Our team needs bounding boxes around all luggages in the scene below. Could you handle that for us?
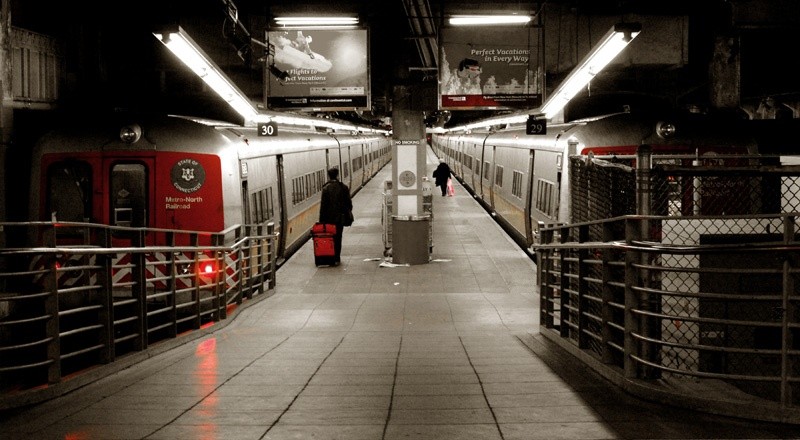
[312,222,337,267]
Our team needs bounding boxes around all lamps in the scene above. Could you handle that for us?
[151,16,394,131]
[423,11,642,134]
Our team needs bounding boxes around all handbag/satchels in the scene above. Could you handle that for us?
[343,210,354,227]
[310,223,336,237]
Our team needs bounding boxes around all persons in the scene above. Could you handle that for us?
[436,159,451,196]
[319,168,353,266]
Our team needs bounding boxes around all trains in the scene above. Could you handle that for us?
[428,115,776,258]
[26,109,388,310]
